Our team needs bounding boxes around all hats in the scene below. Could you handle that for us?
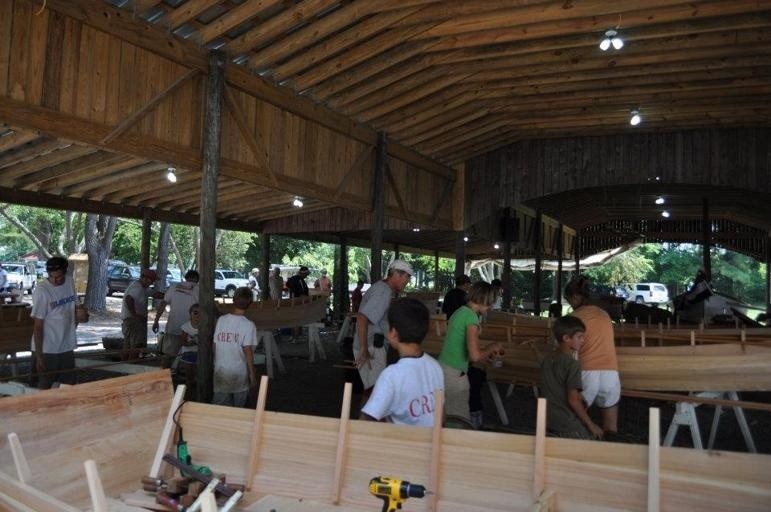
[300,267,310,273]
[251,268,258,273]
[389,260,417,276]
[142,267,156,282]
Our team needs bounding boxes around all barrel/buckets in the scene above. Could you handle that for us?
[177,355,196,399]
[76,309,89,323]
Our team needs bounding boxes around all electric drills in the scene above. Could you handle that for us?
[178,441,212,480]
[368,476,434,512]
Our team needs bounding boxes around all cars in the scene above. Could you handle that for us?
[165,266,190,289]
[613,286,629,299]
[105,263,170,296]
[0,262,38,294]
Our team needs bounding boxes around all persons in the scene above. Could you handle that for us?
[269,266,332,299]
[351,259,507,435]
[152,270,258,408]
[121,269,165,359]
[30,256,89,390]
[537,276,621,441]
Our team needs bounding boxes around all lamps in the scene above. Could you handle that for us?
[596,26,626,54]
[629,104,642,126]
[290,194,307,211]
[167,163,178,187]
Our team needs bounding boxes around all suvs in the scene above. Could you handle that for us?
[214,268,250,299]
[626,281,669,307]
